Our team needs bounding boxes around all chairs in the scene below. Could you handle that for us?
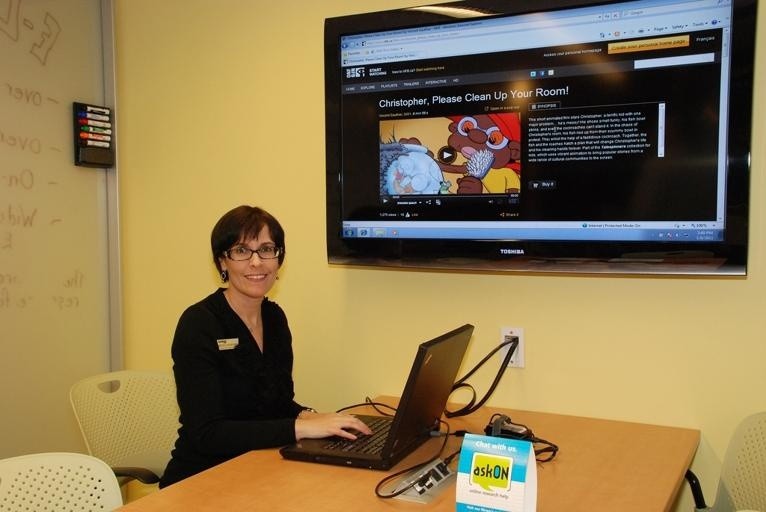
[68,366,183,501]
[686,409,766,510]
[0,452,122,511]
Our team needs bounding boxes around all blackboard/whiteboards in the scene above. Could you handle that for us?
[0,0,137,488]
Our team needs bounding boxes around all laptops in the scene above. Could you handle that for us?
[280,322,476,469]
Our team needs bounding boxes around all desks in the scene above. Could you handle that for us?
[113,392,701,511]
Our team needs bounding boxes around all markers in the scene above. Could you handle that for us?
[77,104,112,148]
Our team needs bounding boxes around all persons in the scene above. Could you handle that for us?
[154,204,372,490]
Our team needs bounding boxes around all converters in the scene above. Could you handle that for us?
[484,419,531,442]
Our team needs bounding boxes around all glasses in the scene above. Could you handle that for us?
[224,245,283,261]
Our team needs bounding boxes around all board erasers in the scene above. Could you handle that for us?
[78,148,114,166]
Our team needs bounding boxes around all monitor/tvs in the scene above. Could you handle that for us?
[323,0,757,278]
